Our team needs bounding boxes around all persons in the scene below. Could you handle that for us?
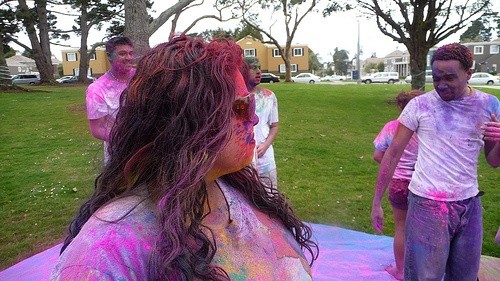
[373,89,426,281]
[49,36,314,281]
[371,43,500,281]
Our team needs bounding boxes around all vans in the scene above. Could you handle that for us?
[361,71,399,84]
[405,69,433,82]
[12,73,41,85]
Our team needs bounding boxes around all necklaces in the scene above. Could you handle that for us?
[108,68,131,83]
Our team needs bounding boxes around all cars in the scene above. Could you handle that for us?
[290,72,321,84]
[56,76,74,83]
[467,71,500,85]
[260,73,281,84]
[61,75,95,83]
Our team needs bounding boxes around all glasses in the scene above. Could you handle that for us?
[232,92,256,123]
